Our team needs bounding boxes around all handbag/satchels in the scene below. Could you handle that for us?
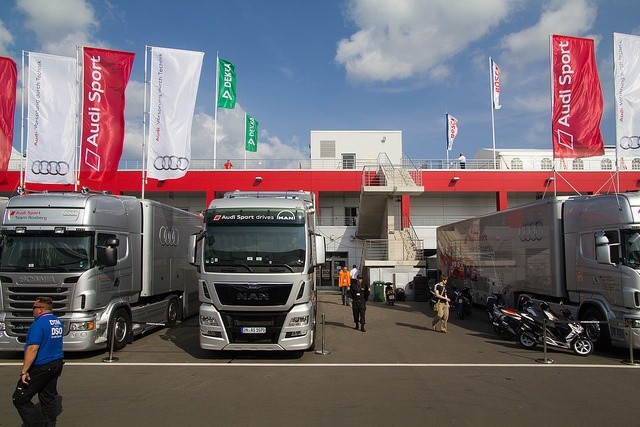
[433,303,439,311]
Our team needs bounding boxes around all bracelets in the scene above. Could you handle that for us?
[19,372,28,375]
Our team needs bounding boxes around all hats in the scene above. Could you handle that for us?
[357,275,363,278]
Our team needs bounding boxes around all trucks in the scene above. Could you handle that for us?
[187,189,326,358]
[436,191,640,349]
[0,185,204,351]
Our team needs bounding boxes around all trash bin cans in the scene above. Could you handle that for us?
[373,281,385,302]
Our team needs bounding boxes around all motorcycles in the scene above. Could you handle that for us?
[487,291,506,318]
[454,285,472,319]
[384,281,397,305]
[515,300,594,356]
[492,297,560,337]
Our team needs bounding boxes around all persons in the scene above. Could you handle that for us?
[224,159,233,168]
[350,264,359,284]
[338,266,351,306]
[458,153,467,168]
[345,274,370,331]
[432,273,451,332]
[12,296,64,426]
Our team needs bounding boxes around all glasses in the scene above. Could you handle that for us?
[33,306,42,309]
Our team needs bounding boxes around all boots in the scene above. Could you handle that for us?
[355,322,359,329]
[361,324,366,332]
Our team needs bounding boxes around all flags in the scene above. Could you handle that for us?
[551,34,605,158]
[0,56,18,183]
[447,114,459,149]
[78,47,136,191]
[492,60,502,109]
[146,46,205,180]
[613,32,640,158]
[27,51,78,191]
[245,112,259,151]
[217,57,236,109]
